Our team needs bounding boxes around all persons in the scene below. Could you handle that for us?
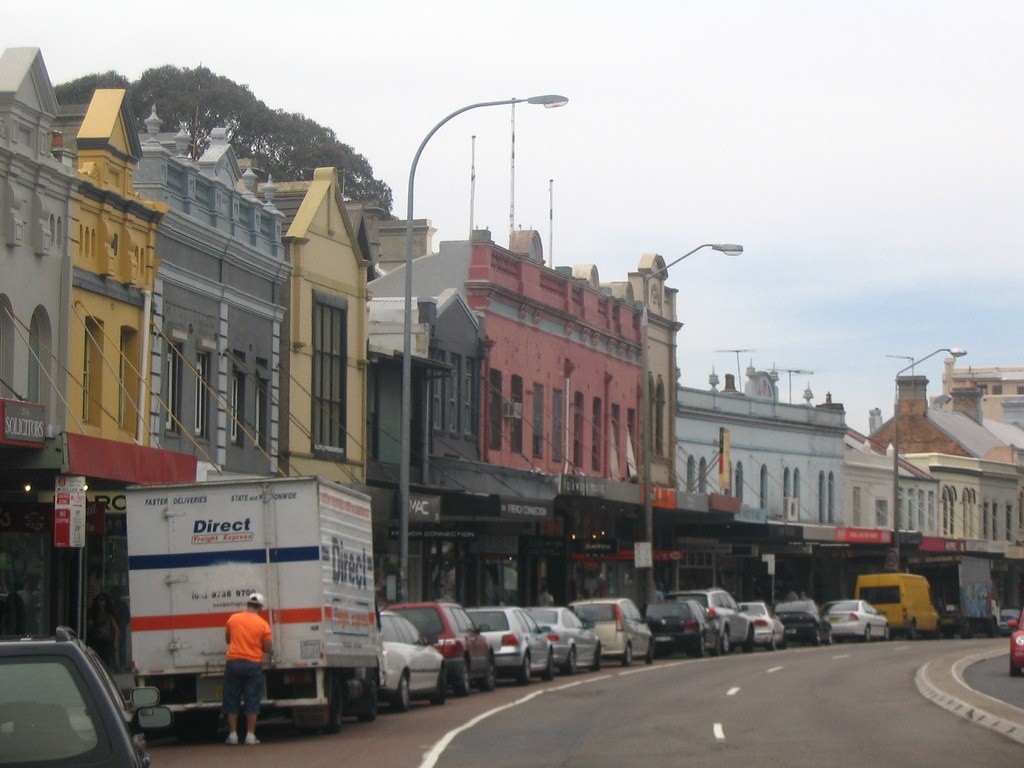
[86,592,120,670]
[535,587,554,607]
[221,592,272,745]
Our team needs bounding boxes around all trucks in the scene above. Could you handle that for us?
[123,476,387,744]
[927,556,999,639]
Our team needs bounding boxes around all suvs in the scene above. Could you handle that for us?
[387,603,498,698]
[0,626,151,768]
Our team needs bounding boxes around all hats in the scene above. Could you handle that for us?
[247,593,263,606]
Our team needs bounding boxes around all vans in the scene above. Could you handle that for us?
[856,573,941,640]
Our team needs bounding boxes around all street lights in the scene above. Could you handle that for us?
[893,348,968,550]
[644,244,743,607]
[885,355,914,375]
[400,95,569,606]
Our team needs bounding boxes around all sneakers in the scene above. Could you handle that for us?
[245,736,260,744]
[226,736,238,744]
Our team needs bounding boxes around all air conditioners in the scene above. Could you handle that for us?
[504,402,522,419]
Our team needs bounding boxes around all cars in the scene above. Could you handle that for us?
[1009,605,1024,678]
[377,610,447,713]
[1000,608,1020,637]
[738,602,788,651]
[642,601,724,658]
[774,599,836,647]
[526,607,602,677]
[467,607,556,686]
[821,600,890,641]
[662,586,754,654]
[568,597,657,668]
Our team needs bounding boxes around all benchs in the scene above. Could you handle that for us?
[0,702,95,764]
